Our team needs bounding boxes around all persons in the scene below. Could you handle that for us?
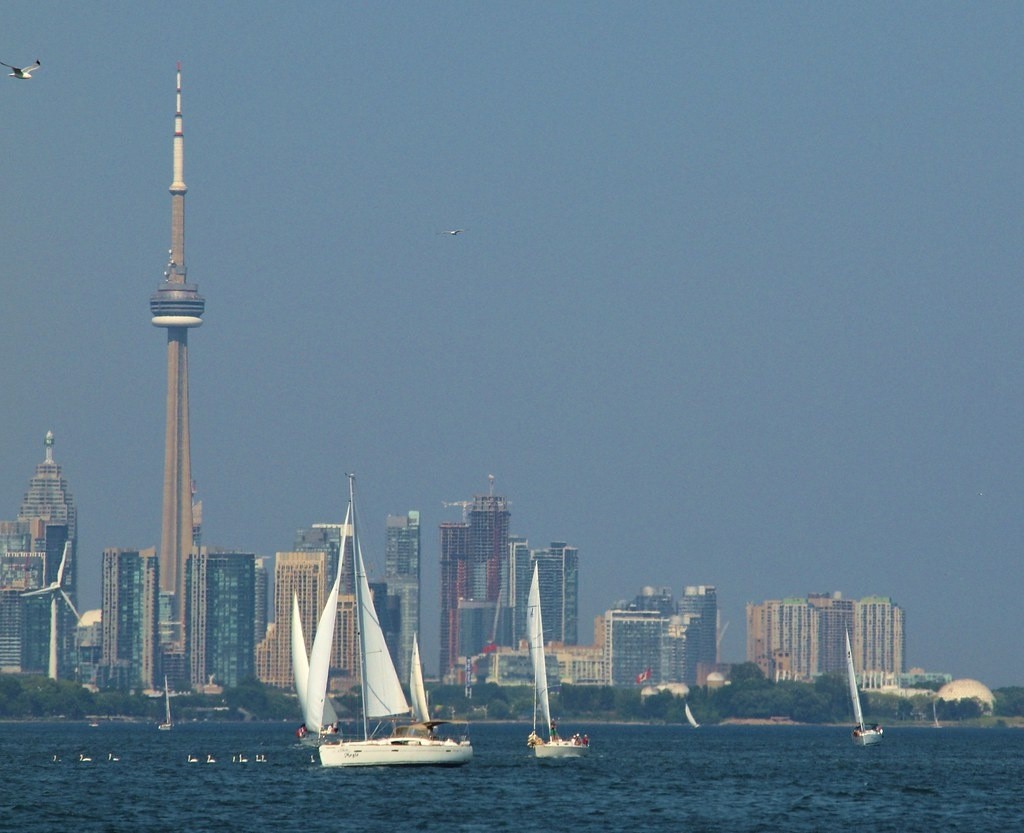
[573,733,589,747]
[550,722,557,741]
[853,724,883,738]
[297,717,338,739]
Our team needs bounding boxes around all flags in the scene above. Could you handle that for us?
[637,668,651,684]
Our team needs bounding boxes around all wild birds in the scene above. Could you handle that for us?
[0,59,40,79]
[436,229,463,236]
[50,753,316,767]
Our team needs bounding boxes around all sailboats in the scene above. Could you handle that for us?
[845,627,883,746]
[290,475,592,768]
[158,675,175,730]
[685,703,700,727]
